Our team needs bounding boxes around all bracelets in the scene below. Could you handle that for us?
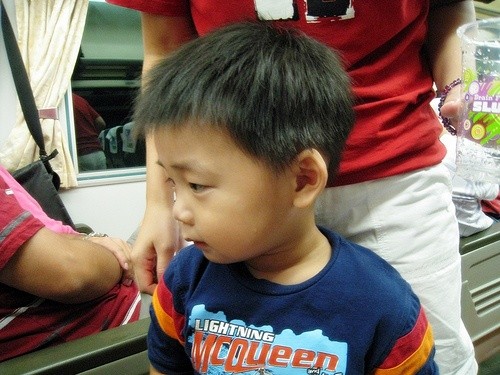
[438,76,468,137]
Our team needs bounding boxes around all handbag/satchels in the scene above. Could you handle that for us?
[15,155,77,231]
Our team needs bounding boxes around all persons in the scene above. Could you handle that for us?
[71,90,108,172]
[1,175,135,301]
[122,17,444,375]
[99,0,500,375]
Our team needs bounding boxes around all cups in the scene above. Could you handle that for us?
[455,17,500,182]
